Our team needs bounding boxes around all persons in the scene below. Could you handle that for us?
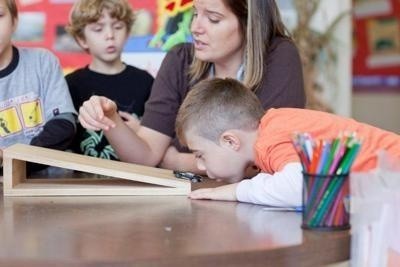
[0,0,79,185]
[64,0,154,174]
[175,77,400,208]
[78,0,306,180]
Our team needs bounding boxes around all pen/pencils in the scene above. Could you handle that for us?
[291,129,366,230]
